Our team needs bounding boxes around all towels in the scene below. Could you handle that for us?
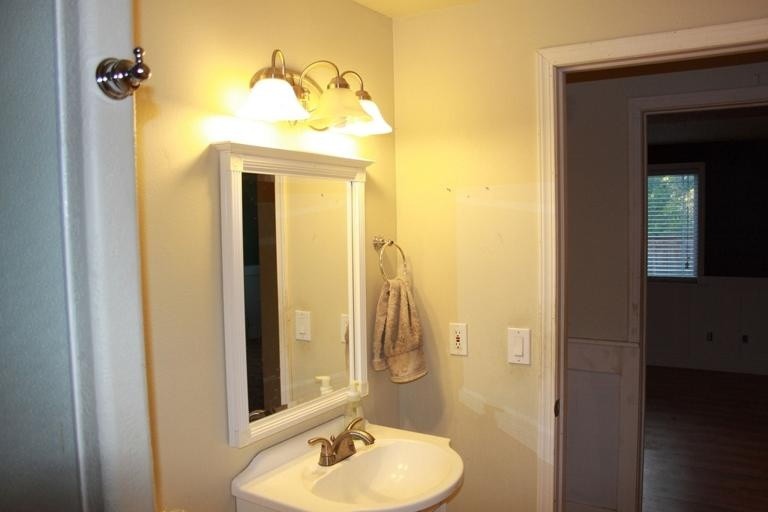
[372,274,431,382]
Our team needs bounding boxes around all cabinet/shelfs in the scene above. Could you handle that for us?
[692,276,741,376]
[646,276,692,370]
[740,276,766,378]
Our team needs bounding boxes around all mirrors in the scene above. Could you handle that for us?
[211,141,377,450]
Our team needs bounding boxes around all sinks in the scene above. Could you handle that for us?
[309,438,453,508]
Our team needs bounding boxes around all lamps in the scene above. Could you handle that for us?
[240,48,393,138]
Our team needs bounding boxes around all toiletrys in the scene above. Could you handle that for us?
[343,381,366,432]
[314,375,334,396]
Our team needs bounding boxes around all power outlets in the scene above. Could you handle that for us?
[448,324,468,357]
[339,313,347,342]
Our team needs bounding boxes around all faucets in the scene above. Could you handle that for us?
[308,417,375,465]
[249,409,273,423]
